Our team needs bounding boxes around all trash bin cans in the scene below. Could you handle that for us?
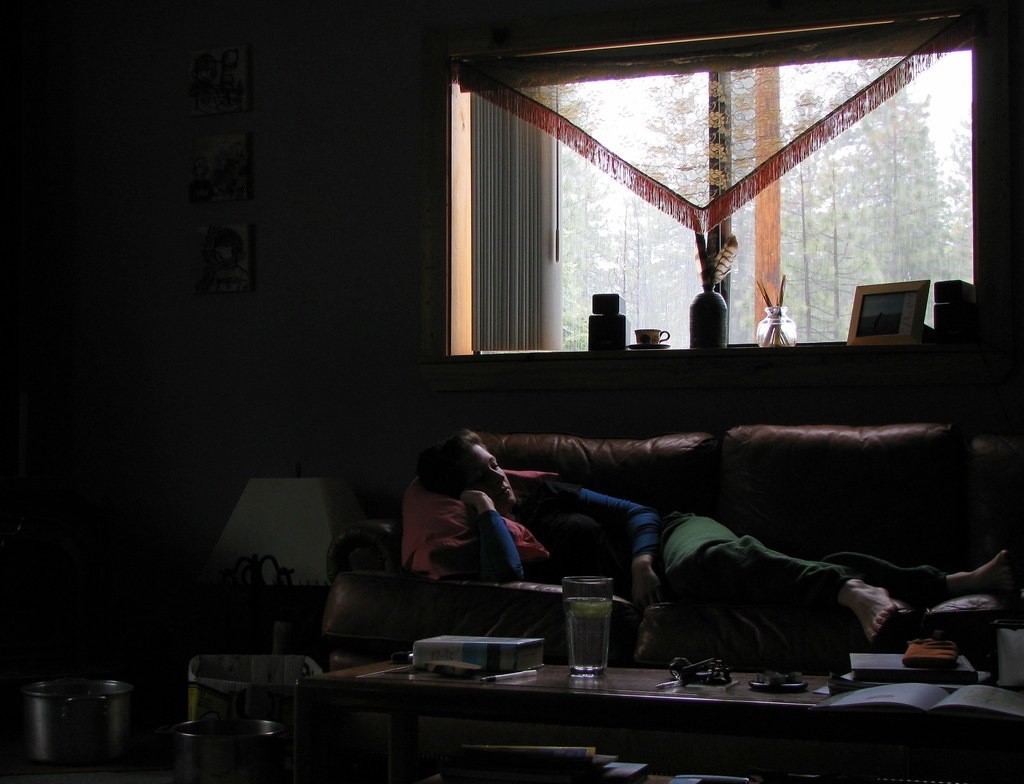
[181,652,322,729]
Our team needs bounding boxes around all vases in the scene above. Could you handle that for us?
[690,283,728,349]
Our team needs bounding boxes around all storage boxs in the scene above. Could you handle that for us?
[186,654,323,784]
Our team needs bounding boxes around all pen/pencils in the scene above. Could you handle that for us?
[654,680,683,692]
[479,668,537,683]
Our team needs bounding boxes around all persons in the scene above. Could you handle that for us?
[415,427,1011,651]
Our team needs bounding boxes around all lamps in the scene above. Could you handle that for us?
[192,461,363,652]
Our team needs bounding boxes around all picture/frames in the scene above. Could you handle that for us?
[847,279,935,345]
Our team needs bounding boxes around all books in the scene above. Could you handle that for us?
[440,744,648,784]
[827,652,994,696]
[747,768,875,784]
[412,635,546,672]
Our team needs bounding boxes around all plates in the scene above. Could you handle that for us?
[629,344,670,349]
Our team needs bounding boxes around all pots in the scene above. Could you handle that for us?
[173,718,289,784]
[25,677,132,765]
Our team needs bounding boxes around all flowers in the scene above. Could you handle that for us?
[688,210,739,284]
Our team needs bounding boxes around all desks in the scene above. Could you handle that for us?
[295,661,1024,784]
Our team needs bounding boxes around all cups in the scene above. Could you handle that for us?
[634,329,670,344]
[563,577,614,675]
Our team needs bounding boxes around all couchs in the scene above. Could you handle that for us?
[320,422,1024,687]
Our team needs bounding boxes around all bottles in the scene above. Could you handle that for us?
[756,308,797,346]
